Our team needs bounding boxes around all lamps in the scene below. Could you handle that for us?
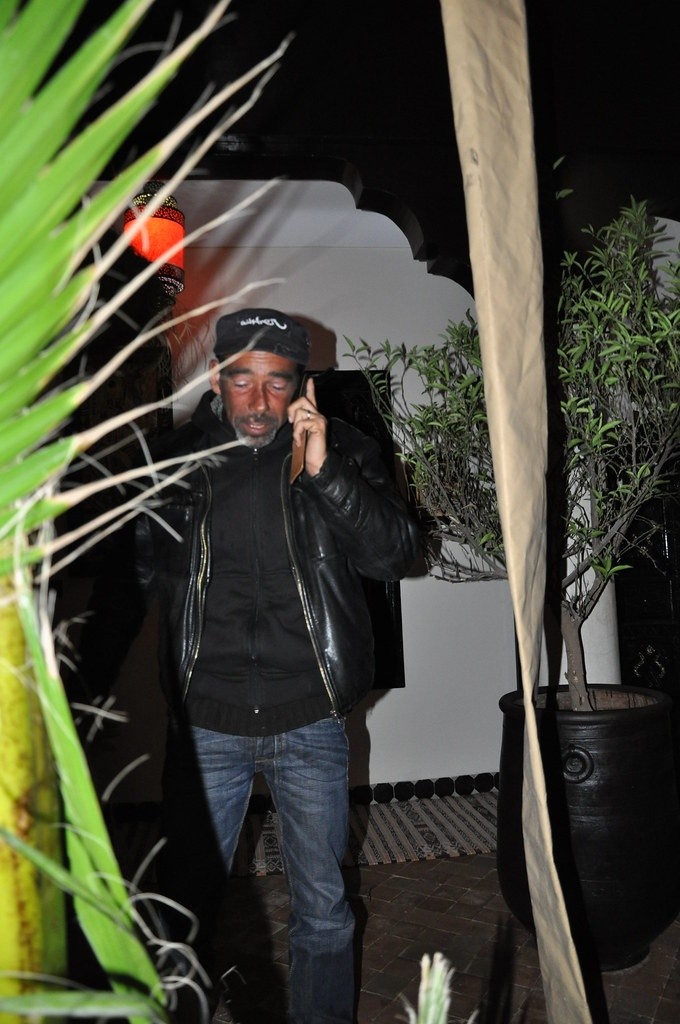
[122,178,185,307]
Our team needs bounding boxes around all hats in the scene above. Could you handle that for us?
[214,308,311,365]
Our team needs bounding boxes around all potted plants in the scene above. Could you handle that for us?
[341,198,680,974]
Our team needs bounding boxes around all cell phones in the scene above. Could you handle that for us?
[293,372,309,402]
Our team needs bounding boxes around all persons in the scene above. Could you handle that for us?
[134,307,417,1024]
